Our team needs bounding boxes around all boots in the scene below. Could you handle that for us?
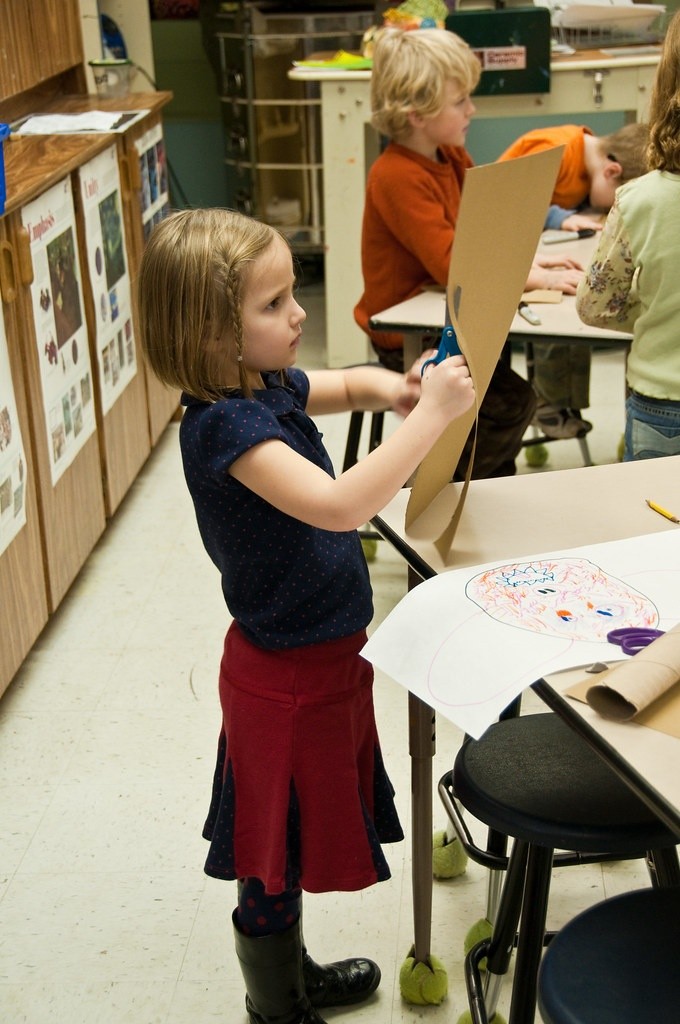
[232,904,327,1024]
[290,887,381,1007]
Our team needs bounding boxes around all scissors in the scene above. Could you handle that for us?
[420,285,462,385]
[607,627,666,656]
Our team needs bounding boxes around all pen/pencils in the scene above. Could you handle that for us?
[645,499,680,523]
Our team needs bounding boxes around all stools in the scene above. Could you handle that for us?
[329,342,597,487]
[437,711,679,1024]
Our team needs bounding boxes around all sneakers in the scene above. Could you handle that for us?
[527,384,593,438]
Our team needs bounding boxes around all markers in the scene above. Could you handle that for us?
[517,300,541,326]
[543,229,596,244]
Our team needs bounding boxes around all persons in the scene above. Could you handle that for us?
[355,28,537,482]
[496,123,653,439]
[574,10,680,462]
[139,207,477,1024]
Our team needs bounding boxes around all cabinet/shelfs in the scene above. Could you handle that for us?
[217,14,389,285]
[0,0,185,702]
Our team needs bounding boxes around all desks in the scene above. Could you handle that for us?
[368,207,635,376]
[367,453,680,1004]
[288,47,661,370]
